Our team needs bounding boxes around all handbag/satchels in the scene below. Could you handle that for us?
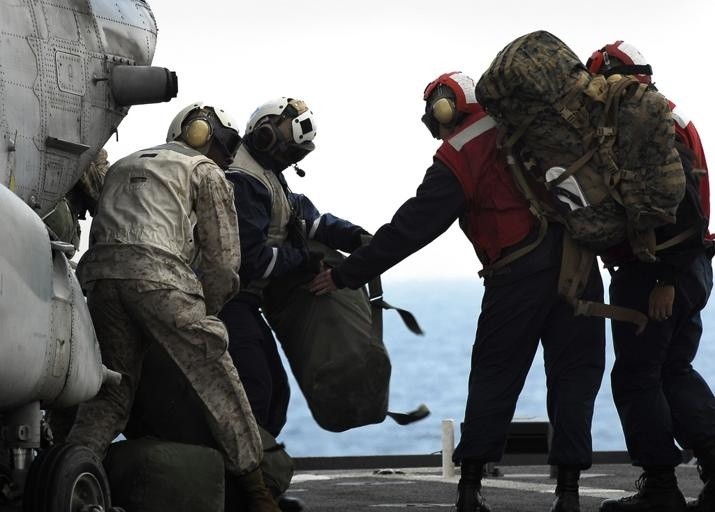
[473,31,686,318]
[260,233,432,433]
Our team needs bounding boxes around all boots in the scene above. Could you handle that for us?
[454,453,492,511]
[548,466,580,512]
[685,452,713,507]
[600,465,686,511]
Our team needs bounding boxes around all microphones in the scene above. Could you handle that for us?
[293,162,305,178]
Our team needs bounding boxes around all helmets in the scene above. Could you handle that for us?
[421,72,480,140]
[166,103,240,170]
[584,41,652,83]
[242,97,318,172]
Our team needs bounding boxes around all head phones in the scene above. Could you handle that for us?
[181,109,214,149]
[432,85,456,125]
[251,107,288,153]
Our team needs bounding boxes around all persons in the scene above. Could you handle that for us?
[64,103,283,511]
[223,95,377,511]
[587,40,715,511]
[307,71,605,511]
